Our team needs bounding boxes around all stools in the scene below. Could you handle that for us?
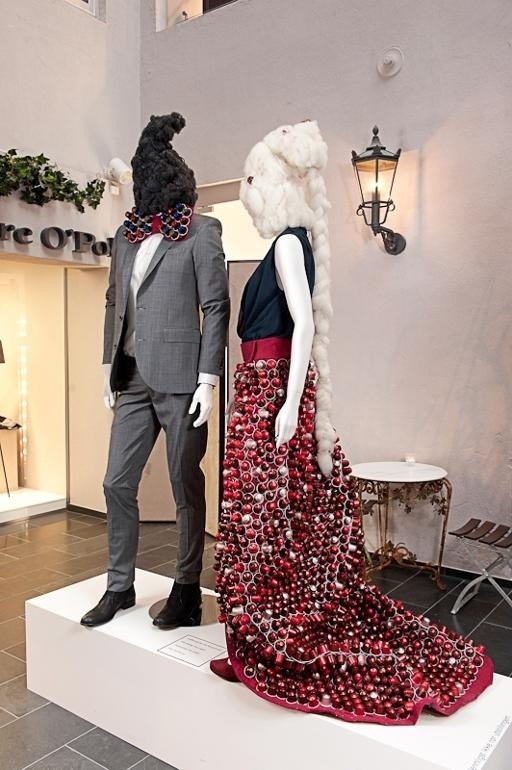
[449,516,511,614]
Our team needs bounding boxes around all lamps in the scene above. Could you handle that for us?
[347,125,405,253]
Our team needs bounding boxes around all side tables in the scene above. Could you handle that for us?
[353,462,452,588]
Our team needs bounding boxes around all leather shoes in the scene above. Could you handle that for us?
[81,589,135,628]
[152,591,203,627]
[211,657,240,682]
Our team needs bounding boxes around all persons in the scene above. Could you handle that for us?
[80,203,231,628]
[209,226,494,728]
